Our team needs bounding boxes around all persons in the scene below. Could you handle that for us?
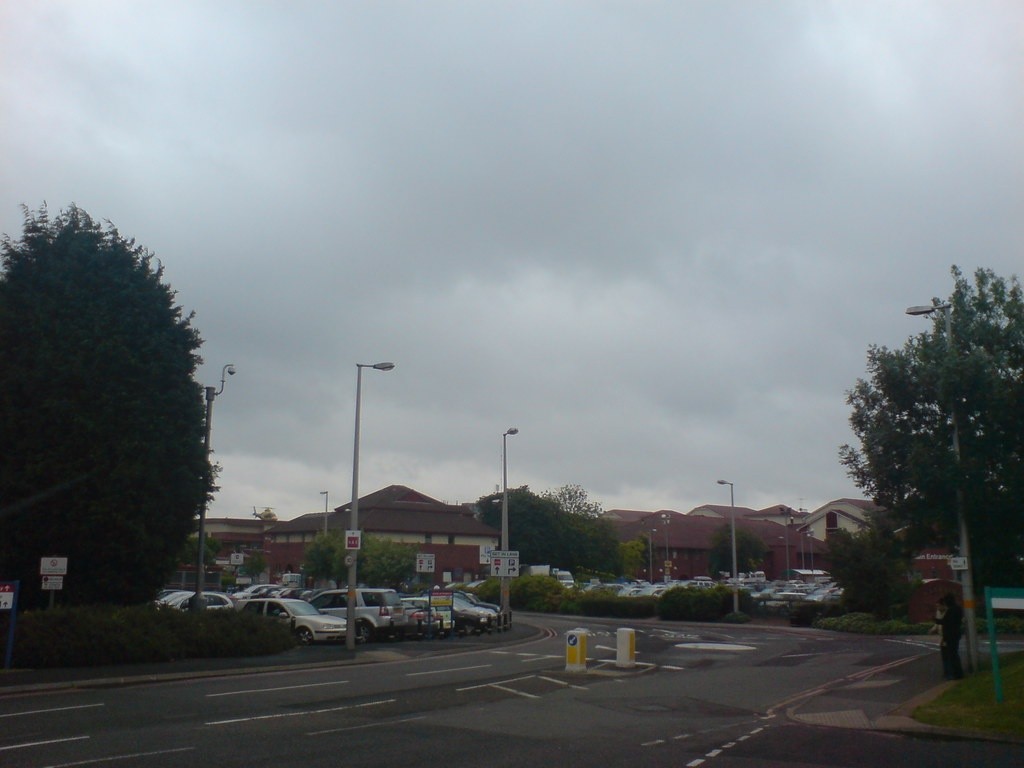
[931,593,964,679]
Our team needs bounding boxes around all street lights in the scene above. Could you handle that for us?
[649,529,657,584]
[320,491,328,538]
[661,513,671,583]
[906,301,979,671]
[189,364,236,612]
[780,507,791,582]
[801,529,814,570]
[500,428,518,630]
[346,363,395,660]
[718,479,738,613]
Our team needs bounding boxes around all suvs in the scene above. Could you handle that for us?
[309,588,400,643]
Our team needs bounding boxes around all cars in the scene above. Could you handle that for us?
[400,591,502,638]
[153,584,321,613]
[234,597,347,644]
[521,565,843,602]
[445,580,488,589]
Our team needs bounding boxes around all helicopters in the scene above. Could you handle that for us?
[252,506,278,521]
